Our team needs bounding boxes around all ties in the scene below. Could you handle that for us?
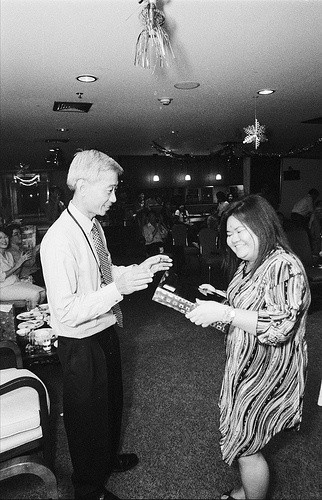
[92,222,124,327]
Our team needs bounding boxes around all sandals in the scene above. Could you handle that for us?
[221,488,238,500]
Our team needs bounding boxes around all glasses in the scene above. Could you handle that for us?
[12,232,22,236]
[0,235,9,241]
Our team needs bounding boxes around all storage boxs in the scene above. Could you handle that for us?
[152,270,230,332]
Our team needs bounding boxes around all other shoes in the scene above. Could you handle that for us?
[74,489,122,500]
[312,263,322,268]
[179,271,190,276]
[111,453,139,473]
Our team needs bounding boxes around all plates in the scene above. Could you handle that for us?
[16,304,58,346]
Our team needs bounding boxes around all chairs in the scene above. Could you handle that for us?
[199,228,226,281]
[283,226,322,316]
[169,224,195,266]
[0,347,57,500]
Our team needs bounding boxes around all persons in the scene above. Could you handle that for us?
[141,210,169,283]
[44,185,65,216]
[146,191,162,204]
[174,187,233,248]
[0,224,46,308]
[185,196,311,500]
[39,150,174,500]
[291,188,322,251]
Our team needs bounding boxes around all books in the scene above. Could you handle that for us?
[152,270,231,334]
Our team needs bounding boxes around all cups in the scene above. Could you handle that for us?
[24,331,35,353]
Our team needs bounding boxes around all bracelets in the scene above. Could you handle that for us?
[223,307,235,324]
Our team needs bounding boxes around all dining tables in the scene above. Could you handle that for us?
[14,306,57,372]
